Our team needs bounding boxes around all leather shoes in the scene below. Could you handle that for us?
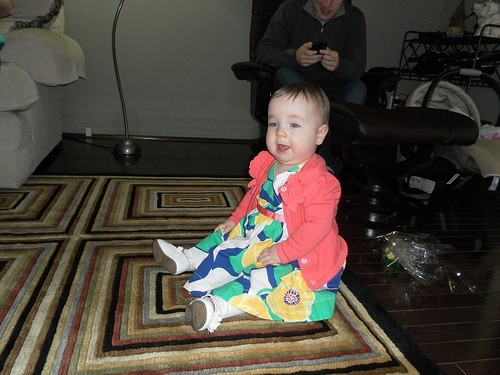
[152,238,190,276]
[185,293,227,332]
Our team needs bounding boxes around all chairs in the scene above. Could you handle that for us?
[228,0,400,150]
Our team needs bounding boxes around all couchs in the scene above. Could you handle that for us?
[0,26,87,189]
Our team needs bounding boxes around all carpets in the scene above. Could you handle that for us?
[0,174,417,375]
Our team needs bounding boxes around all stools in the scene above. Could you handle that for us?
[358,106,480,199]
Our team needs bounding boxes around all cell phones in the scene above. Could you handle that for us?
[309,42,329,55]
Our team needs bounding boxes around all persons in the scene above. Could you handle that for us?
[153,79,348,333]
[255,0,366,174]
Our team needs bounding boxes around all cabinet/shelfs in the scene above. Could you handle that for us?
[390,12,500,109]
